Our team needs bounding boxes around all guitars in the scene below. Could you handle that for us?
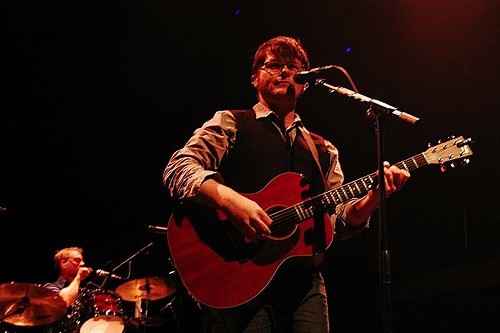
[166,135,473,315]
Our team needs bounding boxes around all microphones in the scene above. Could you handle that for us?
[293,66,338,85]
[96,269,122,279]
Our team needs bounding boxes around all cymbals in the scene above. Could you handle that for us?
[115,277,175,301]
[0,282,68,326]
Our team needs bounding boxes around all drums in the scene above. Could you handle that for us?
[72,288,125,333]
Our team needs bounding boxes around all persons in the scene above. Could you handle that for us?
[43,247,93,307]
[162,36,410,333]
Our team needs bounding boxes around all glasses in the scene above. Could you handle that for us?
[258,59,306,76]
